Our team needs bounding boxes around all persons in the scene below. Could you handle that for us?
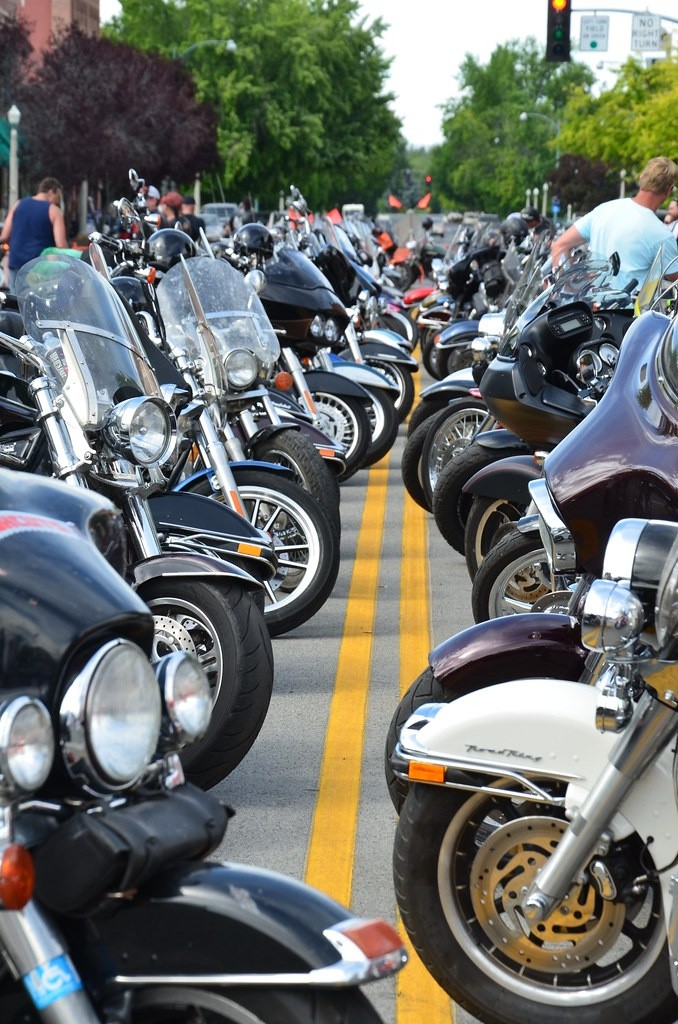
[222,199,255,238]
[145,186,205,241]
[522,207,557,241]
[0,177,70,292]
[552,156,678,310]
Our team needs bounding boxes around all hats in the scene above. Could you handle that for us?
[146,187,161,200]
[521,207,538,221]
[163,192,184,208]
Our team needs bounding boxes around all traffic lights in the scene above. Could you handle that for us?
[546,0,571,62]
[425,176,432,187]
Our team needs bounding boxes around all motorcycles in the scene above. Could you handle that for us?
[0,167,425,1024]
[401,212,678,1024]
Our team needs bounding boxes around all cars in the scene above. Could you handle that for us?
[203,203,237,222]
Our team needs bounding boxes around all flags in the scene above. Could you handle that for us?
[389,194,402,208]
[418,193,431,208]
[377,231,394,250]
[290,209,313,229]
[326,208,342,224]
[388,248,411,264]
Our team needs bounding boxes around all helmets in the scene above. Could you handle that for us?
[233,224,275,257]
[501,219,528,244]
[145,231,196,268]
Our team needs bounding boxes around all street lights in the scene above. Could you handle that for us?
[7,105,21,212]
[520,112,561,222]
